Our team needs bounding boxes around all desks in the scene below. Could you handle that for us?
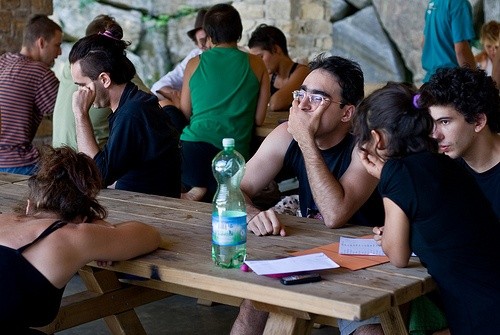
[0,169,439,335]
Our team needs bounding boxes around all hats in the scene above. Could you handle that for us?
[187,9,207,41]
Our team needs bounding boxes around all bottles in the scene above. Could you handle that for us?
[210,138,247,268]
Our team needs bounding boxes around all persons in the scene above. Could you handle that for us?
[172,4,277,201]
[230,55,385,335]
[0,14,62,175]
[409,66,500,334]
[248,24,310,111]
[0,146,160,335]
[420,0,500,82]
[52,14,185,200]
[150,8,212,101]
[351,84,500,335]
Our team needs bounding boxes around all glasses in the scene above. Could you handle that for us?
[292,90,342,106]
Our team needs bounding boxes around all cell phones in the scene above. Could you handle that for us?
[280,273,321,285]
